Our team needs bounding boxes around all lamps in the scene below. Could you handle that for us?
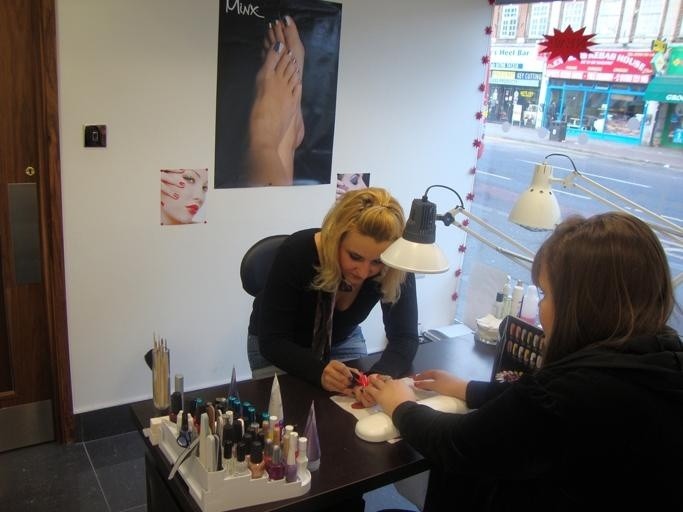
[377,184,537,289]
[510,154,683,288]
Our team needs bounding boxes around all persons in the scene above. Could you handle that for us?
[249,186,419,511]
[242,16,306,187]
[160,168,208,227]
[364,209,683,509]
[546,100,557,130]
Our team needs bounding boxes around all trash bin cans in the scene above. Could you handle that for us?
[549,121,567,142]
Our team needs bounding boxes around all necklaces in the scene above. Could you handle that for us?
[555,96,566,121]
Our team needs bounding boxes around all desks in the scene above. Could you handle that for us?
[129,332,526,512]
